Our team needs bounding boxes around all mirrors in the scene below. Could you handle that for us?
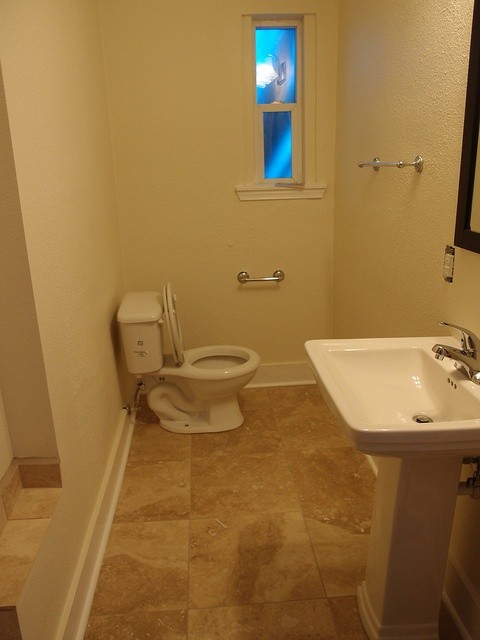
[454,0,480,253]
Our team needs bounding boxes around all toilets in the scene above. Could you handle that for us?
[117,282,260,434]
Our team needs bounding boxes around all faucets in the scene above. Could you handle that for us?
[431,321,480,385]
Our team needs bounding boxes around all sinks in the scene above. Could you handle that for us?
[304,335,480,455]
